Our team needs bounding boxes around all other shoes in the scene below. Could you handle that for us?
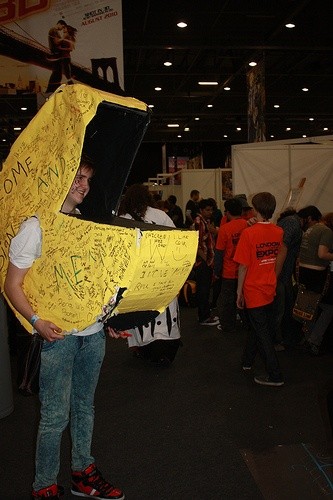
[239,361,252,370]
[303,343,333,354]
[254,374,284,387]
[275,337,290,351]
[199,316,220,325]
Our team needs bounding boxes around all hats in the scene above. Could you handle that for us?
[241,199,253,208]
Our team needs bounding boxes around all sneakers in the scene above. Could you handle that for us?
[70,464,125,500]
[33,483,66,500]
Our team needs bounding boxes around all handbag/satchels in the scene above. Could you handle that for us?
[16,333,42,396]
[291,286,323,326]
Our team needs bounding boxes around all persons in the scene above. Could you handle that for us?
[4,156,125,500]
[115,178,333,385]
[46,20,79,95]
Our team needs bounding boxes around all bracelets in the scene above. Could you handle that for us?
[29,315,39,326]
